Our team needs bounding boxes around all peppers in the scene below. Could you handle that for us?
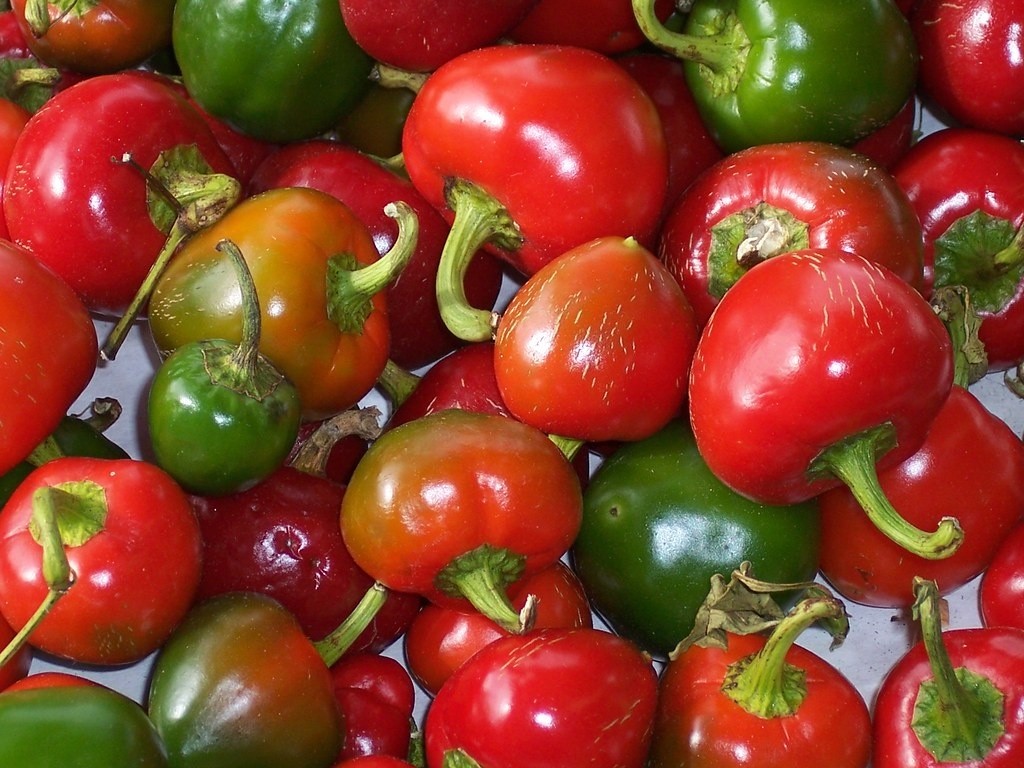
[0,0,1024,768]
